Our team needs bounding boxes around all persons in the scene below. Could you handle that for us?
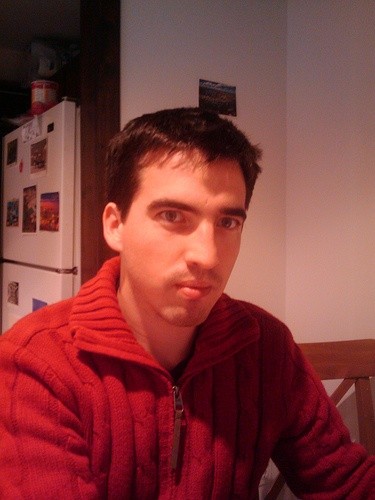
[0,106,375,500]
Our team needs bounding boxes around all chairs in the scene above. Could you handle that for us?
[248,339,375,500]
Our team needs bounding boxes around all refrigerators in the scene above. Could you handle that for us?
[0,105,119,331]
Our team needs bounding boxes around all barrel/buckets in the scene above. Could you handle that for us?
[30,79,58,115]
